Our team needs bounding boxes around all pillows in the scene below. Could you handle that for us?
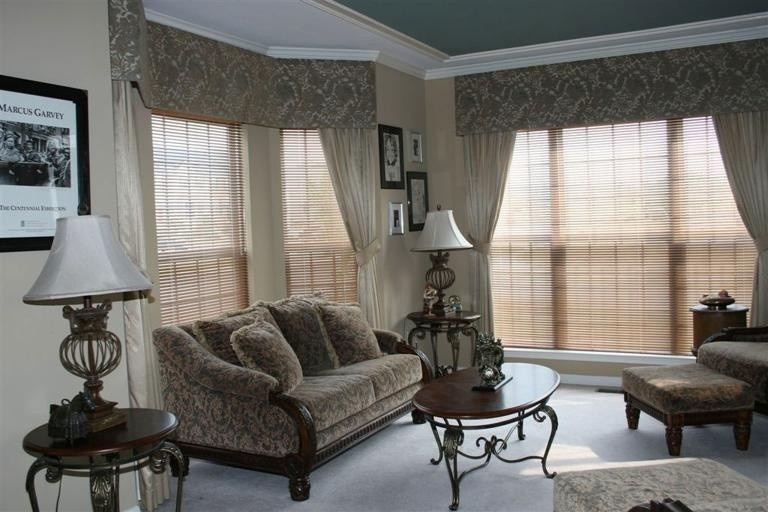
[312,300,387,363]
[230,314,303,398]
[195,301,283,379]
[261,296,339,374]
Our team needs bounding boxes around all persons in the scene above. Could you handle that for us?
[0,130,71,188]
[385,134,398,167]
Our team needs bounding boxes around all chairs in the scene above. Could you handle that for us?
[551,458,768,512]
[697,323,767,413]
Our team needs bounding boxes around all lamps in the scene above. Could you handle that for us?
[407,201,475,315]
[21,214,155,429]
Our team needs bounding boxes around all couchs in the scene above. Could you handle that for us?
[149,290,435,501]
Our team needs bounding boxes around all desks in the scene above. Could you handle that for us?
[21,404,188,511]
[688,303,749,357]
[406,308,481,378]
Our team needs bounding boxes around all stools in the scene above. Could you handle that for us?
[618,361,758,458]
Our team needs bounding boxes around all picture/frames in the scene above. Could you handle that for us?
[0,73,92,252]
[404,171,431,233]
[386,199,404,237]
[376,121,405,190]
[409,131,423,166]
